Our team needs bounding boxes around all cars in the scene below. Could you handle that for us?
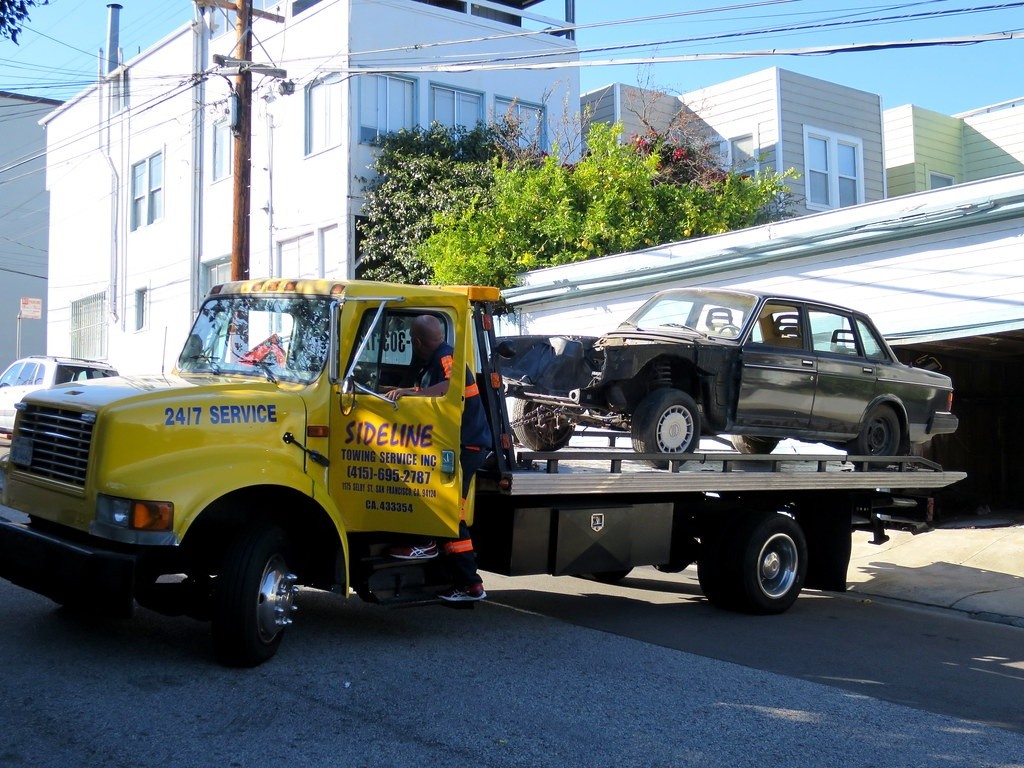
[489,285,957,470]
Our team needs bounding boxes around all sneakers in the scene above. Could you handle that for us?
[436,581,487,602]
[381,541,439,559]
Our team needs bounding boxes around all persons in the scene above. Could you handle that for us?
[377,314,492,603]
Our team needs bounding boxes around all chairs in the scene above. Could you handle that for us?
[708,308,860,353]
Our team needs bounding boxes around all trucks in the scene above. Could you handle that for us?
[0,276,938,670]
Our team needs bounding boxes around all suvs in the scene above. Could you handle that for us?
[0,355,120,442]
